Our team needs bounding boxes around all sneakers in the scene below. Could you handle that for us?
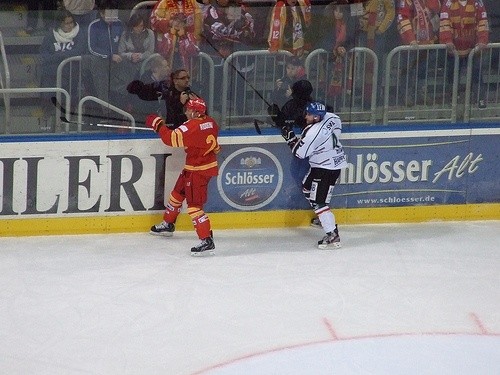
[318,224,341,250]
[149,219,176,239]
[310,217,323,228]
[190,230,216,257]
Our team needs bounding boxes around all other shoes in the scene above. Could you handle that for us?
[417,97,435,106]
[474,97,487,111]
[402,95,414,107]
[449,96,460,106]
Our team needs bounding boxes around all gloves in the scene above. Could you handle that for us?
[145,114,163,132]
[267,104,285,127]
[279,123,301,156]
[127,79,146,96]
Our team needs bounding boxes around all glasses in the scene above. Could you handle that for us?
[173,76,191,80]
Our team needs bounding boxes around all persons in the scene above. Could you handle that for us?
[39,0,489,130]
[281,102,348,249]
[145,97,221,257]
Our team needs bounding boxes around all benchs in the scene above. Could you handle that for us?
[246,55,500,92]
[0,10,50,133]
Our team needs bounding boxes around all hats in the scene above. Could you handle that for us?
[286,54,301,65]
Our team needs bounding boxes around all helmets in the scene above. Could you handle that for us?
[183,99,207,116]
[305,101,326,122]
[291,80,314,97]
